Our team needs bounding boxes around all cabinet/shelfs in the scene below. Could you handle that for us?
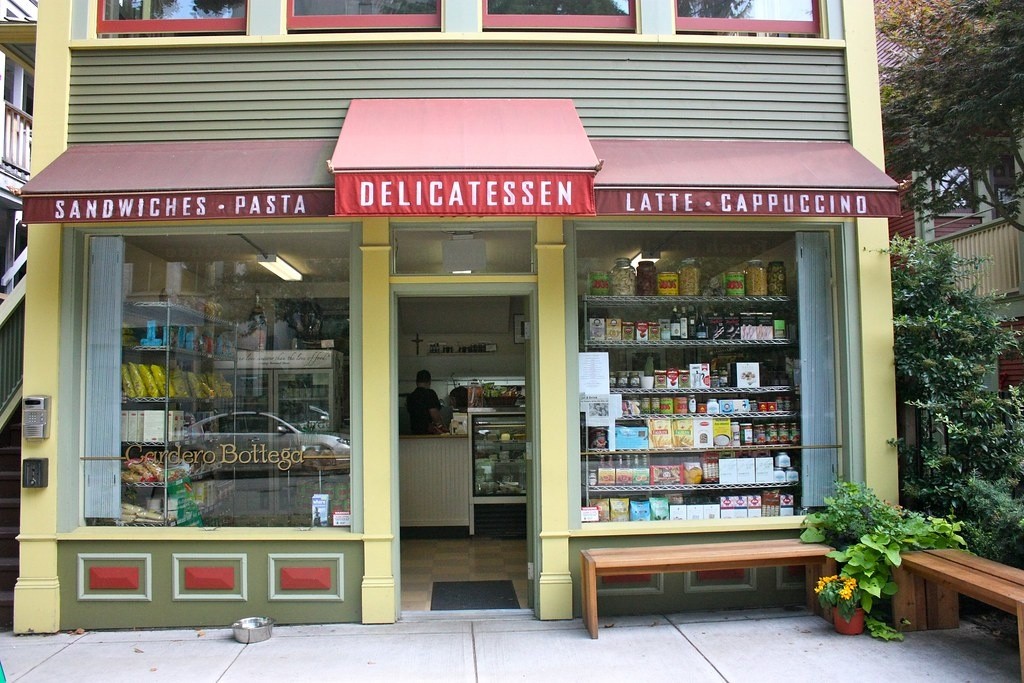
[121,296,239,527]
[579,292,803,523]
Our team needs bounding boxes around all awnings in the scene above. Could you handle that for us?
[330,99,601,216]
[590,140,903,218]
[20,140,338,222]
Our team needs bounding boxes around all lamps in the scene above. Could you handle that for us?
[258,252,301,281]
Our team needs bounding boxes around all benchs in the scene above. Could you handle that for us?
[580,538,836,639]
[890,547,1024,683]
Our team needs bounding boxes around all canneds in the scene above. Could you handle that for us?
[609,371,707,414]
[586,270,611,296]
[722,271,745,295]
[281,387,329,398]
[656,272,679,296]
[740,423,801,445]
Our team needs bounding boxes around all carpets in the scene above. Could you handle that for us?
[431,580,521,611]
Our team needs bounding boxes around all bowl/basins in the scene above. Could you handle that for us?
[231,616,276,644]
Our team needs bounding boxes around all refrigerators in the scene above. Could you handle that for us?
[213,351,344,515]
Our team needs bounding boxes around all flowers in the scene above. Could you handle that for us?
[814,575,861,624]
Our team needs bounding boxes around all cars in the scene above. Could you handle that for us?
[179,411,351,480]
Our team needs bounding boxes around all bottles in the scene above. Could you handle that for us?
[680,307,688,340]
[670,306,680,340]
[430,343,485,353]
[695,315,708,340]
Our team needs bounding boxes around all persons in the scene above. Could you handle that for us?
[407,370,449,435]
[449,387,467,413]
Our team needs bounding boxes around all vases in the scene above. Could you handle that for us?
[832,605,865,635]
